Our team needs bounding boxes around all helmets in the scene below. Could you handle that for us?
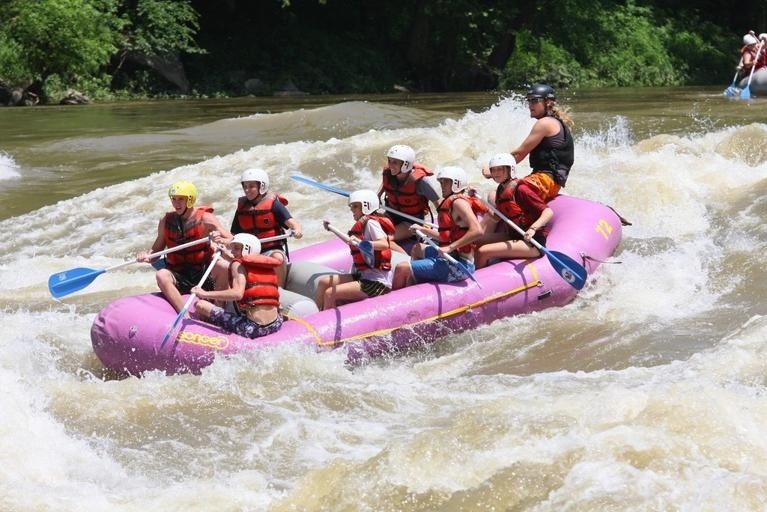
[348,189,379,215]
[230,233,261,257]
[387,145,415,173]
[168,182,197,208]
[240,168,269,195]
[743,34,756,46]
[436,166,469,193]
[527,84,556,100]
[488,152,516,179]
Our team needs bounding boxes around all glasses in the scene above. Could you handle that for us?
[527,98,544,103]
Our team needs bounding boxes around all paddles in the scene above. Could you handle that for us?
[474,192,586,289]
[322,222,375,268]
[152,232,293,270]
[722,57,743,97]
[402,221,483,290]
[291,175,439,229]
[160,248,222,351]
[48,236,210,298]
[740,40,765,98]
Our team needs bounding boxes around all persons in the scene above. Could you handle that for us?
[735,29,767,76]
[216,168,303,292]
[482,83,575,209]
[315,189,396,312]
[392,166,485,291]
[376,143,443,241]
[136,180,233,320]
[473,154,554,272]
[190,233,284,339]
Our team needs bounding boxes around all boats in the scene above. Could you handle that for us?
[91,196,622,373]
[739,66,767,95]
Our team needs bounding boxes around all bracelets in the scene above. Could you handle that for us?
[449,243,456,251]
[529,225,537,231]
